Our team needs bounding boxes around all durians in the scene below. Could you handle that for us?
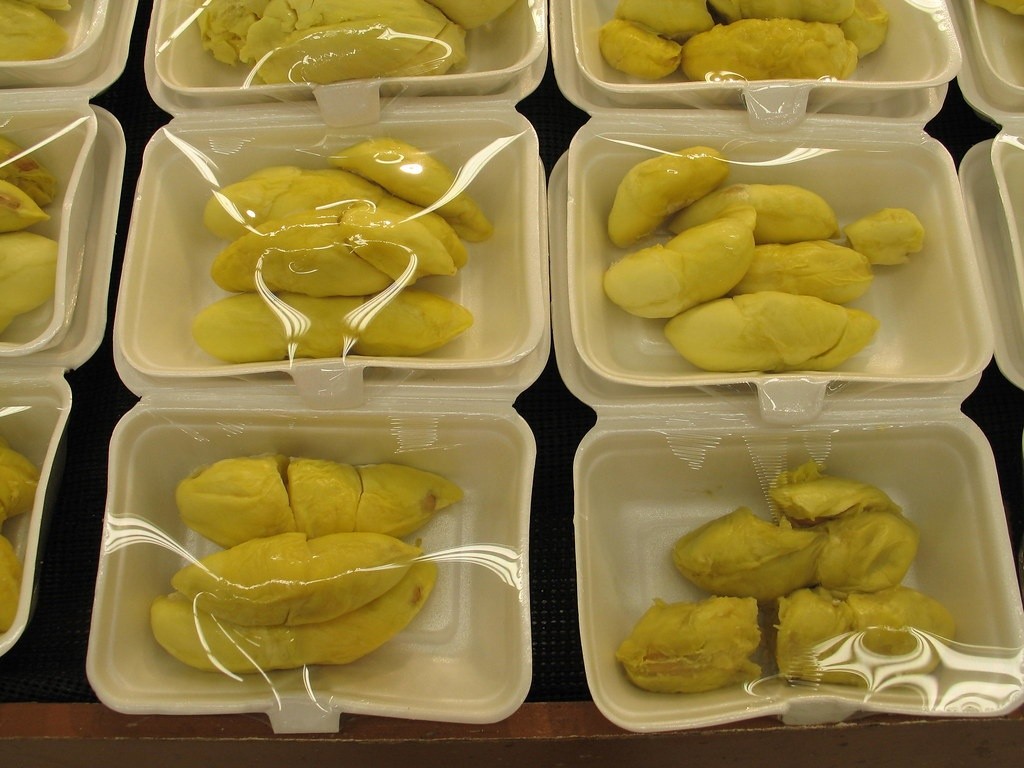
[0,0,955,694]
